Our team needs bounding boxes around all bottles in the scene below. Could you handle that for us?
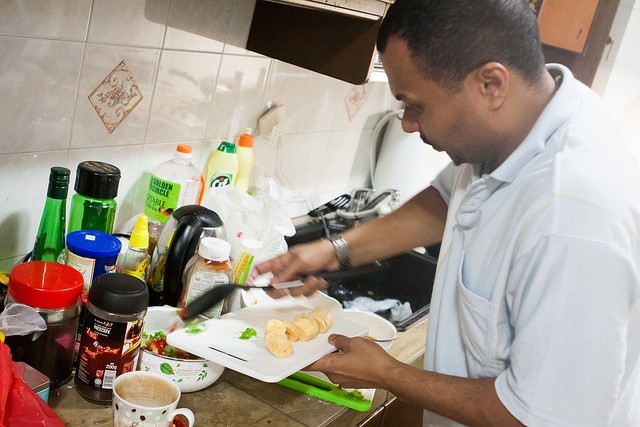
[28,167,71,264]
[235,133,255,188]
[203,141,238,189]
[118,212,150,282]
[146,143,207,252]
[67,161,121,235]
[182,236,231,318]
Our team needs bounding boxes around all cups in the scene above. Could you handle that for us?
[112,371,194,427]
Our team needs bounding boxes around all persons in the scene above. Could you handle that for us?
[243,1,638,426]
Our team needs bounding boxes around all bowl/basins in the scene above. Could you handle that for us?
[139,307,227,393]
[343,308,399,353]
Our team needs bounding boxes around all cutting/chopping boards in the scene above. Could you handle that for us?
[166,306,371,383]
[274,370,373,413]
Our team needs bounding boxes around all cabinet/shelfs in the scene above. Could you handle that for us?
[530,0,601,55]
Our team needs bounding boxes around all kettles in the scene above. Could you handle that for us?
[147,204,226,306]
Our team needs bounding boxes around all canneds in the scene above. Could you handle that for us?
[66,229,121,291]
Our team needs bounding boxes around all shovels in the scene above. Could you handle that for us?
[179,262,392,323]
